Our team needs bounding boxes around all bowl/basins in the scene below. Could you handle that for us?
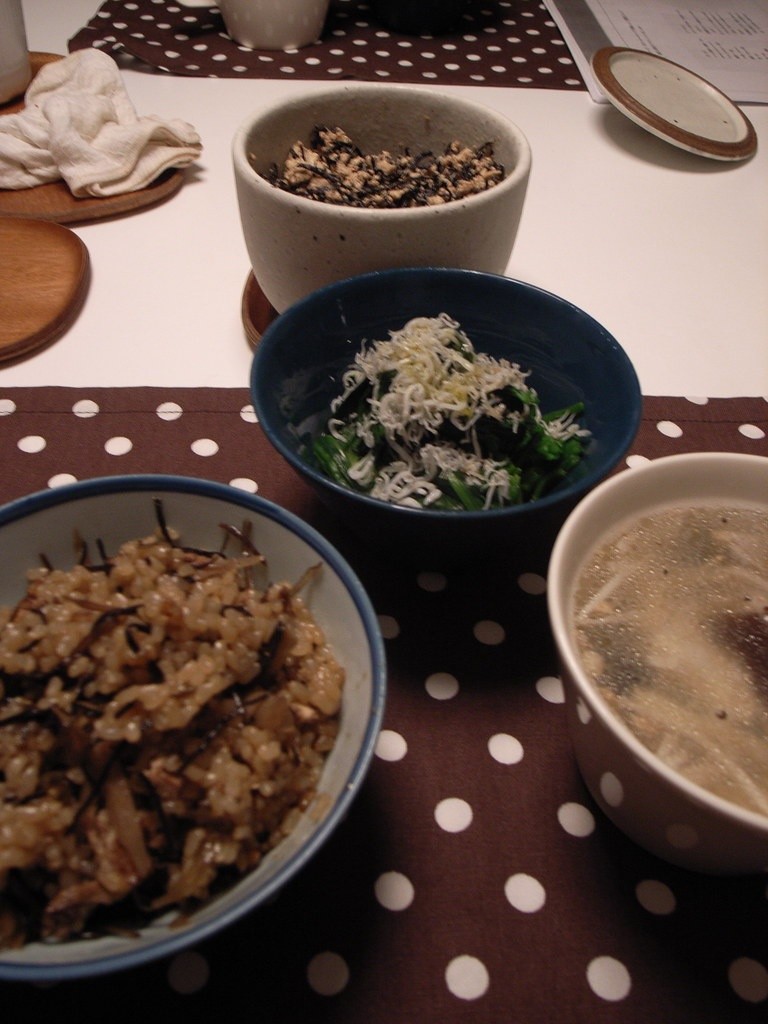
[546,451,768,876]
[249,267,643,549]
[235,90,531,316]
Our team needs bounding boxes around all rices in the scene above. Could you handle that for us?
[0,526,345,947]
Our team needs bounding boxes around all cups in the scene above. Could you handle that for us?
[216,0,329,51]
[0,0,31,110]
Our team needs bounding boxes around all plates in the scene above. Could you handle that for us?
[0,472,387,983]
[241,269,281,354]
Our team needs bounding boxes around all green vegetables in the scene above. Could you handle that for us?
[311,370,590,510]
